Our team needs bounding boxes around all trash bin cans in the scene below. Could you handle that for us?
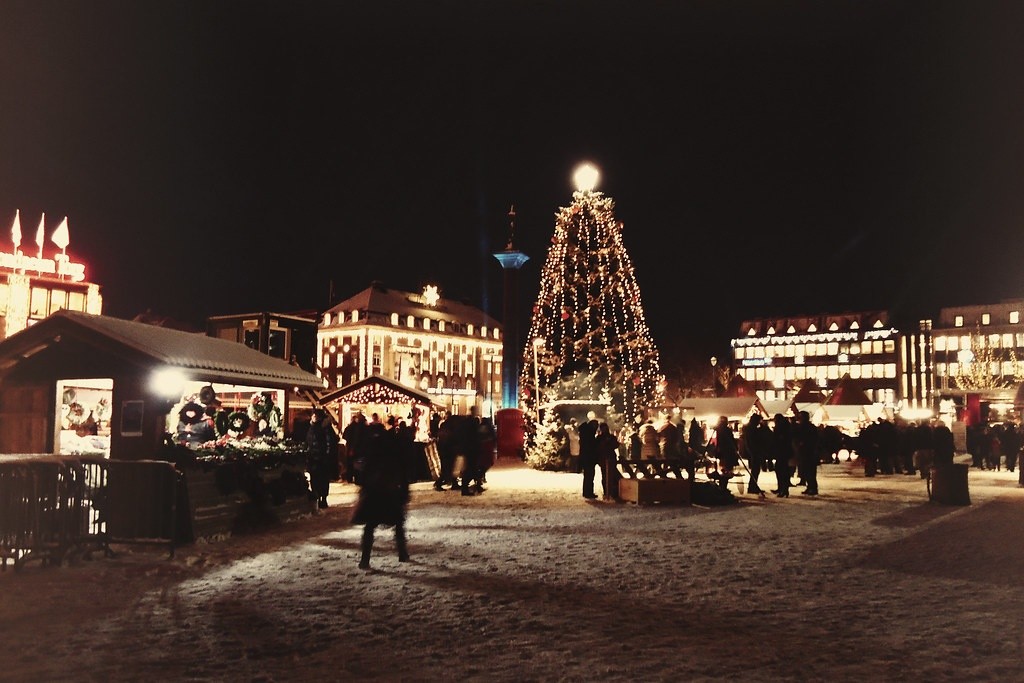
[929,462,972,506]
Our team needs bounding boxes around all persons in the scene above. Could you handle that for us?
[555,391,821,502]
[822,417,1023,485]
[292,396,498,570]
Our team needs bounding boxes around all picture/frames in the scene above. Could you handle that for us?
[120,400,144,436]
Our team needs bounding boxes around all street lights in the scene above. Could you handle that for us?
[711,357,717,398]
[489,348,497,429]
[532,336,546,425]
[956,349,973,408]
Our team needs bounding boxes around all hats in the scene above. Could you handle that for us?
[311,410,328,423]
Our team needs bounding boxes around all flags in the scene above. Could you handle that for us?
[51,220,69,249]
[11,212,22,247]
[35,217,44,248]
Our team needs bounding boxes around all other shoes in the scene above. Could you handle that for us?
[583,492,598,498]
[801,487,818,495]
[747,488,765,493]
[434,487,447,491]
[796,481,806,486]
[788,483,796,487]
[450,485,461,490]
[473,487,484,492]
[461,492,474,496]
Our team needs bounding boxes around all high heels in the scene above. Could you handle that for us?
[776,492,789,498]
[770,488,780,494]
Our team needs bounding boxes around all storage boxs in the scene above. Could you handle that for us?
[619,478,684,505]
